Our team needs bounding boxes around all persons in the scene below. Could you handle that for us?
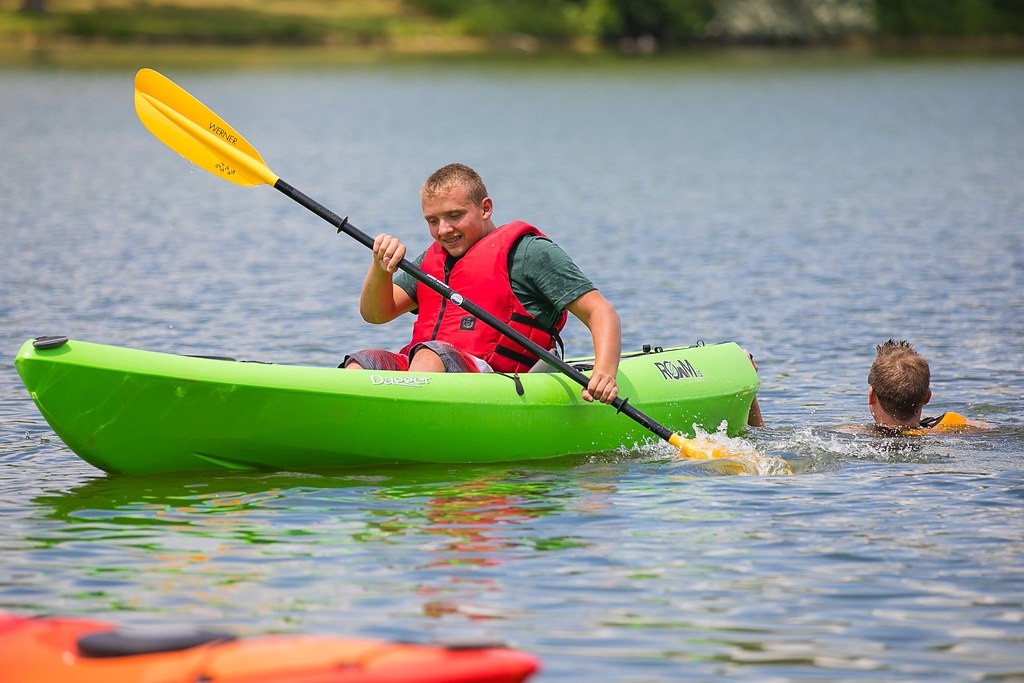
[745,338,995,429]
[339,164,622,406]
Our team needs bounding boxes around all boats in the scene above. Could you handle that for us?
[0,610,539,683]
[12,335,758,476]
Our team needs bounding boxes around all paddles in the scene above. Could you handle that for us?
[133,67,728,460]
